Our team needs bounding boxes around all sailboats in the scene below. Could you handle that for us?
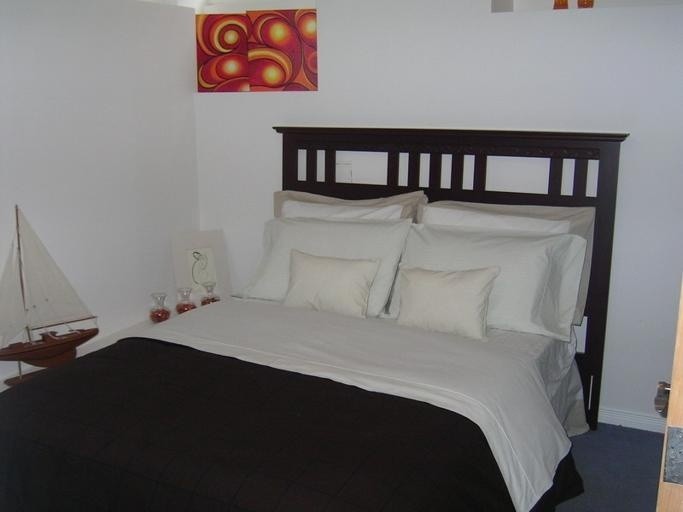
[1,205,100,363]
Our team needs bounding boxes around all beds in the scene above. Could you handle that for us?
[0,126,629,512]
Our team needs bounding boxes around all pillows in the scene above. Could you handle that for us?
[239,191,430,320]
[386,200,596,339]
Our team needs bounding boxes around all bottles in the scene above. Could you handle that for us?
[201,283,220,306]
[175,288,197,314]
[150,292,170,322]
